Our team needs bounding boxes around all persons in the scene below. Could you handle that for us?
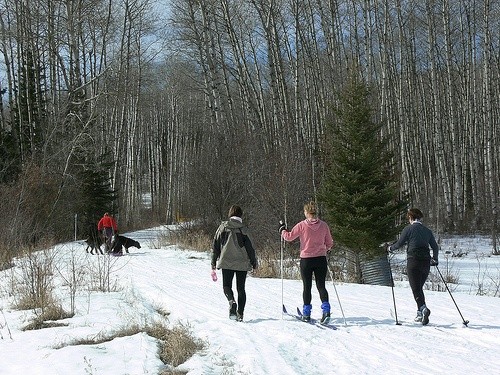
[98,212,118,253]
[279,202,334,325]
[211,204,258,321]
[388,208,439,326]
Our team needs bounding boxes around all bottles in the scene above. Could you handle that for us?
[211,270,217,281]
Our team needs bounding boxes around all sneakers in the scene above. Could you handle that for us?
[300,315,310,322]
[414,311,423,322]
[420,306,431,325]
[321,313,330,324]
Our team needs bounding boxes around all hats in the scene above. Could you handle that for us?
[228,205,242,217]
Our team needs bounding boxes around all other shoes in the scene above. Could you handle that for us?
[229,300,237,320]
[234,311,243,322]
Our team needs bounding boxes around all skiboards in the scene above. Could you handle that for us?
[282,305,351,333]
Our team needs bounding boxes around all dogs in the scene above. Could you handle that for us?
[85,234,141,256]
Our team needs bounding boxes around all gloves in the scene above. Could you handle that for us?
[387,246,393,254]
[278,225,286,234]
[430,256,439,266]
